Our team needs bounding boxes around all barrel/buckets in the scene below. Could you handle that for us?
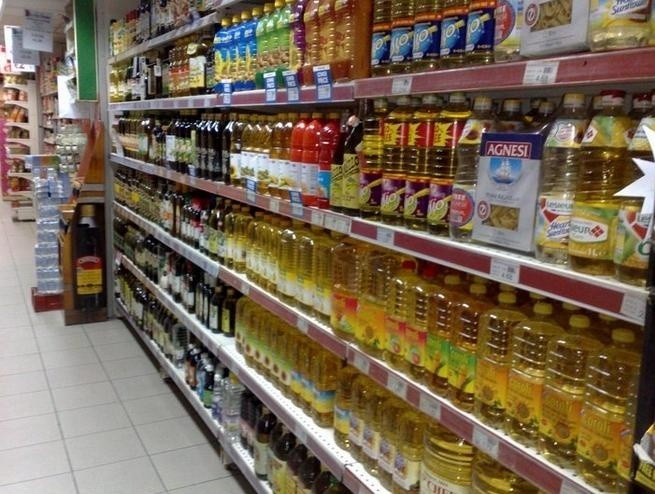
[107,1,655,493]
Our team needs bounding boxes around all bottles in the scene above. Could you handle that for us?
[107,0,655,494]
[74,203,106,311]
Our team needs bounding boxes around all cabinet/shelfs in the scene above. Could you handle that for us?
[2,0,655,493]
